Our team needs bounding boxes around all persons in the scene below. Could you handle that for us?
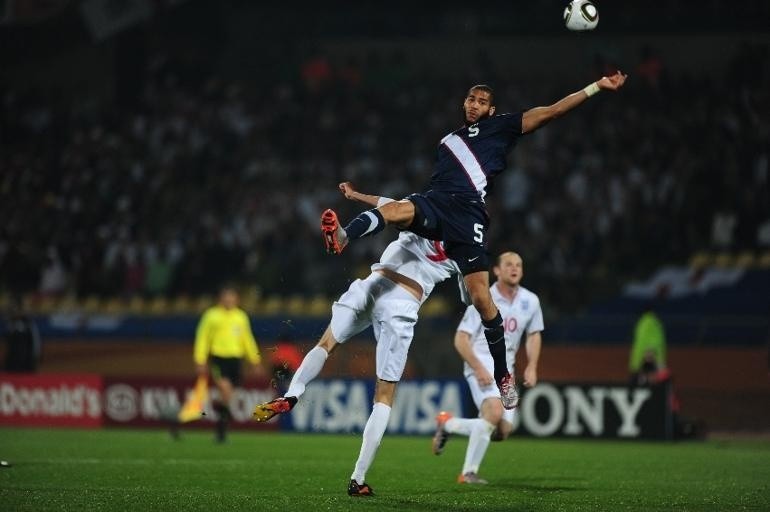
[253,181,471,496]
[321,69,629,408]
[0,1,770,444]
[431,249,545,483]
[192,283,261,447]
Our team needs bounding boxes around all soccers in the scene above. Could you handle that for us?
[564,0,600,31]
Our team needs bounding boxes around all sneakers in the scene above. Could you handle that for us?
[253,397,298,424]
[494,374,519,409]
[320,209,349,253]
[431,411,451,455]
[348,479,374,497]
[457,470,487,485]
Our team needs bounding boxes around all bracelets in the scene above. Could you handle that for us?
[584,83,599,97]
